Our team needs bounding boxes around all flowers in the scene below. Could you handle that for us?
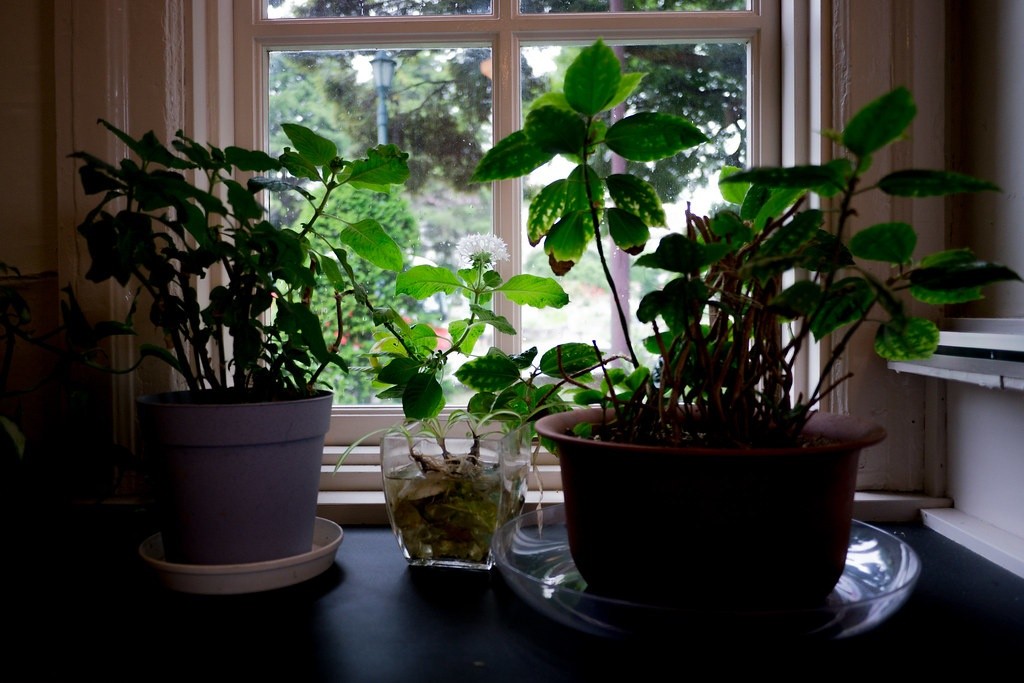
[333,403,545,561]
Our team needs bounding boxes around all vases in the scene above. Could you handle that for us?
[377,413,533,571]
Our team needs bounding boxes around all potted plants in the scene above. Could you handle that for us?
[0,113,570,595]
[462,34,1024,644]
